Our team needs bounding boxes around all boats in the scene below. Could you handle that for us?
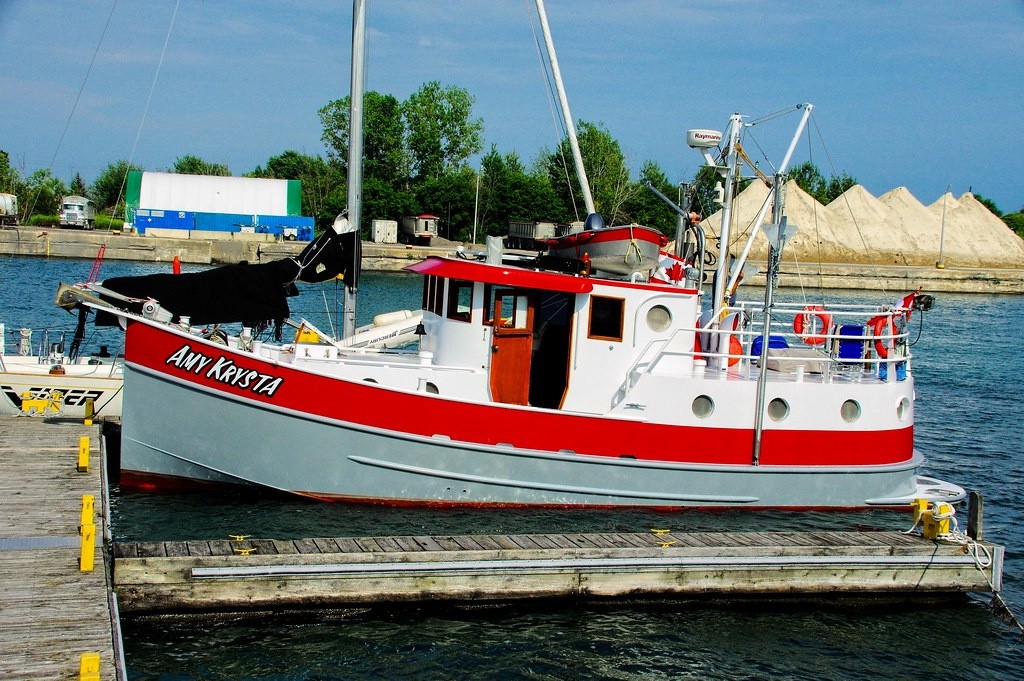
[44,1,972,517]
[0,351,124,419]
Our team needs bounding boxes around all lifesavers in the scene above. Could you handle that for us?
[874,317,898,358]
[794,306,834,344]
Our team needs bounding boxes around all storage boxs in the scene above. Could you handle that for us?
[283,227,298,237]
[406,245,412,249]
[241,226,254,233]
[124,222,132,228]
[122,228,130,233]
[112,230,120,235]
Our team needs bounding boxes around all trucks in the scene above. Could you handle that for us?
[57,195,96,229]
